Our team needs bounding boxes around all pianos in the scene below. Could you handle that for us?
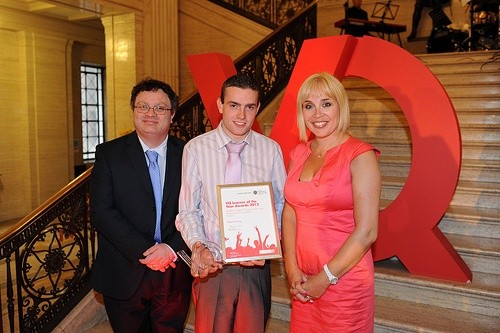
[334,18,407,34]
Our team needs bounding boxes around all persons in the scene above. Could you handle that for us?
[175,74,287,332]
[89,78,192,333]
[281,73,382,333]
[345,0,375,36]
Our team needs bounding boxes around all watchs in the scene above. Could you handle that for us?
[323,263,338,285]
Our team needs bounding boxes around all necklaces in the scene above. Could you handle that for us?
[318,143,333,158]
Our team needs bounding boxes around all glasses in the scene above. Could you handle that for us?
[134,104,172,115]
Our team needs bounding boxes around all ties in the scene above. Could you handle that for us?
[145,150,163,243]
[224,141,247,184]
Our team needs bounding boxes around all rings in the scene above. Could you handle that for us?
[160,259,164,264]
[152,264,157,268]
